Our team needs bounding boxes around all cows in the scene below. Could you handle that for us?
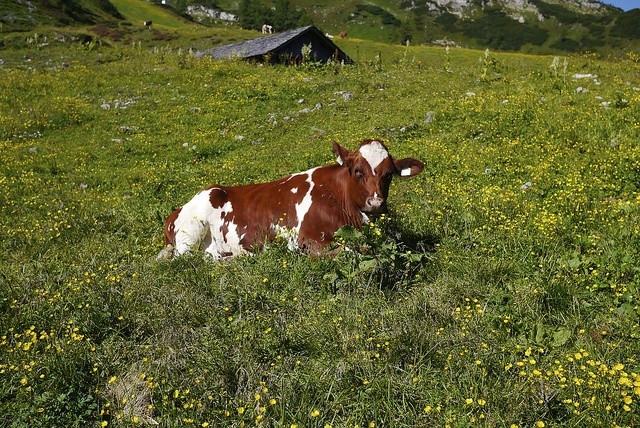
[143,20,152,29]
[155,140,424,261]
[262,24,274,34]
[340,32,346,38]
